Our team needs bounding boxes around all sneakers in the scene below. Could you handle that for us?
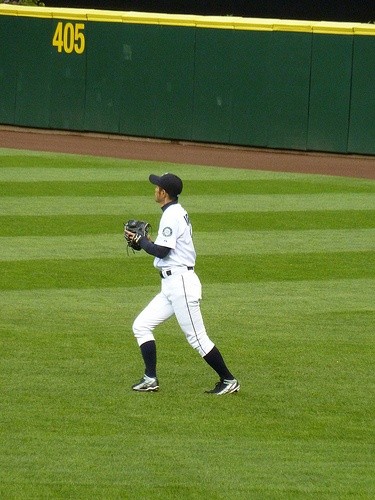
[130,374,159,391]
[204,378,240,395]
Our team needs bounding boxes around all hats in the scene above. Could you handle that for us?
[148,172,183,195]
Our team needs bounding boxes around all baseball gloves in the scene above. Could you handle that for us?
[124,219,151,255]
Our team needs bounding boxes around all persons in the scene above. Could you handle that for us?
[125,173,241,395]
